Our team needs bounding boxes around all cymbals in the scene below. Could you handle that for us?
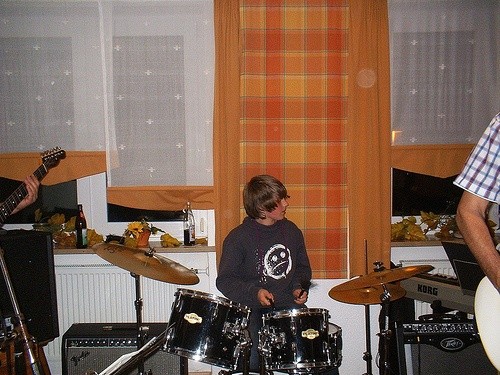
[94,243,200,286]
[334,266,435,291]
[328,282,406,305]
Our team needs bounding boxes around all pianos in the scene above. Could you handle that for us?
[399,272,474,314]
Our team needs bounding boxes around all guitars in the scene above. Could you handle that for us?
[474,275,500,372]
[0,146,66,228]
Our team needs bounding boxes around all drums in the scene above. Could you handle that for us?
[262,308,330,370]
[162,288,250,370]
[329,322,343,369]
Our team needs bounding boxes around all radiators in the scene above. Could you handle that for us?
[402,260,474,320]
[43,266,194,359]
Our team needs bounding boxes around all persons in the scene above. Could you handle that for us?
[0,173,39,216]
[216,174,340,375]
[453,111,500,294]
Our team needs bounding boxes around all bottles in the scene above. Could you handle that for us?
[75,204,88,249]
[183,201,195,246]
